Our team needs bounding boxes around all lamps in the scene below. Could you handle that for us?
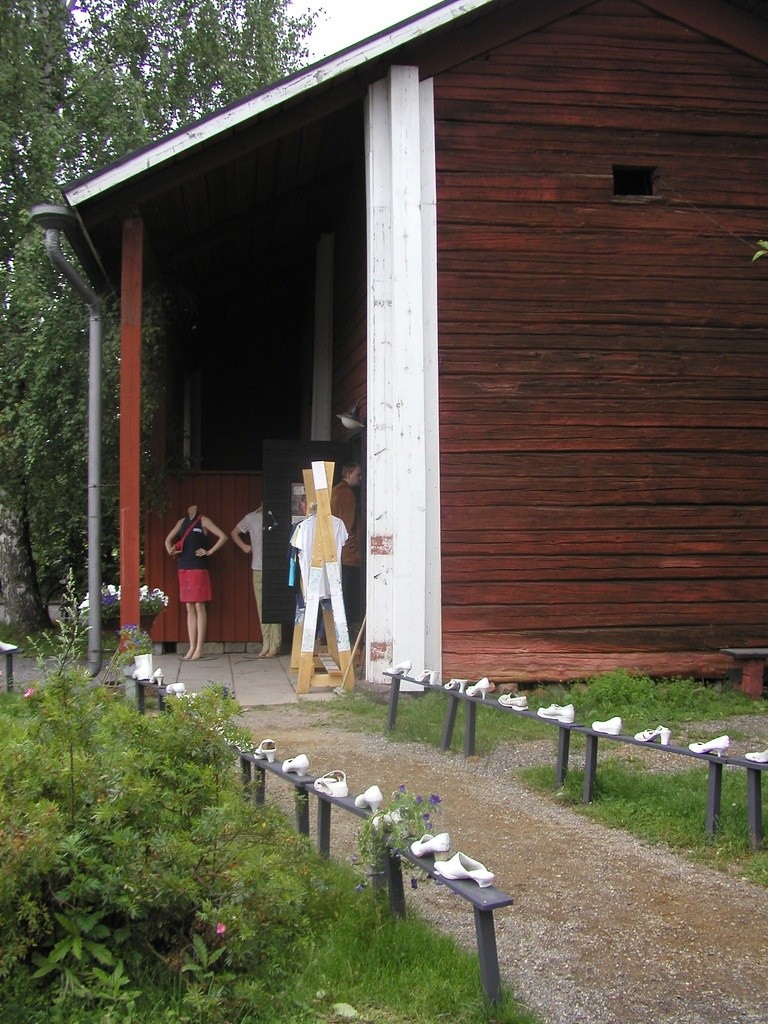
[337,406,365,429]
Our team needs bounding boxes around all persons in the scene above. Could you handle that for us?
[164,505,228,659]
[317,461,362,645]
[230,502,282,658]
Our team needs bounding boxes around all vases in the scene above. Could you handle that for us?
[104,680,126,700]
[111,613,155,636]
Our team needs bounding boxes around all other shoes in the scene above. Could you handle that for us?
[745,749,768,762]
[318,636,326,644]
[592,717,622,735]
[372,808,410,838]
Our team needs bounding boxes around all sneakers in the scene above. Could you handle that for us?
[537,704,574,723]
[498,692,528,711]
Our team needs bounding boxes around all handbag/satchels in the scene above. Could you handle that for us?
[173,540,183,553]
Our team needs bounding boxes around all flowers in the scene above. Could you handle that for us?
[114,623,153,654]
[78,585,169,620]
[349,785,443,891]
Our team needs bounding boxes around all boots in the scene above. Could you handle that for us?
[132,654,153,680]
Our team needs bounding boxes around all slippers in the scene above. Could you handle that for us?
[313,770,348,797]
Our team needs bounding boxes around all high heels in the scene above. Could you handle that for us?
[150,668,163,687]
[411,833,450,861]
[634,725,671,745]
[415,670,440,685]
[354,785,382,815]
[254,739,277,763]
[386,661,412,678]
[434,852,495,888]
[444,678,467,694]
[689,735,729,757]
[466,677,490,700]
[282,754,309,777]
[166,683,186,699]
